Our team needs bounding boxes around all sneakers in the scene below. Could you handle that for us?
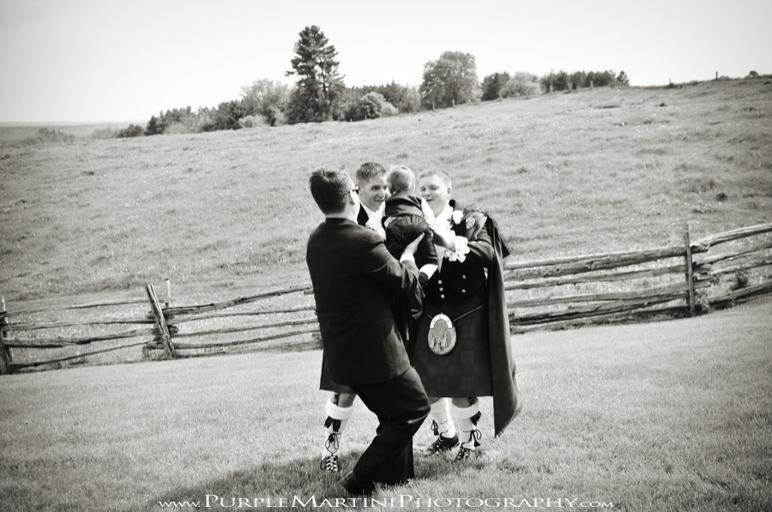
[426,431,460,454]
[318,453,340,473]
[455,443,482,462]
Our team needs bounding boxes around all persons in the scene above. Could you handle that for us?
[306,163,524,495]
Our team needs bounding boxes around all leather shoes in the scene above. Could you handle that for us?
[336,469,375,499]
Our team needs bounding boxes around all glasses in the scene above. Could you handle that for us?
[340,183,362,201]
